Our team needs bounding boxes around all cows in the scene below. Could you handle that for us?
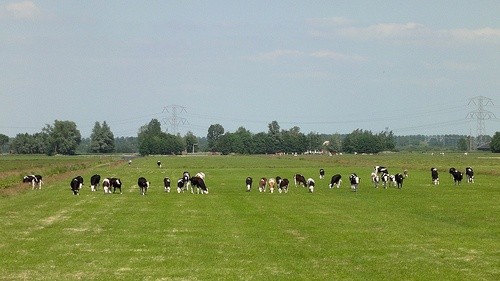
[465,167,474,183]
[23,174,44,190]
[431,167,440,185]
[176,171,209,195]
[245,177,252,192]
[258,177,268,193]
[293,173,315,193]
[449,167,463,185]
[267,177,276,194]
[69,175,84,196]
[128,161,132,167]
[370,165,408,190]
[328,173,342,189]
[319,168,325,180]
[89,173,101,192]
[156,160,161,167]
[137,176,152,196]
[348,173,361,192]
[276,176,290,194]
[102,177,122,194]
[163,177,172,193]
